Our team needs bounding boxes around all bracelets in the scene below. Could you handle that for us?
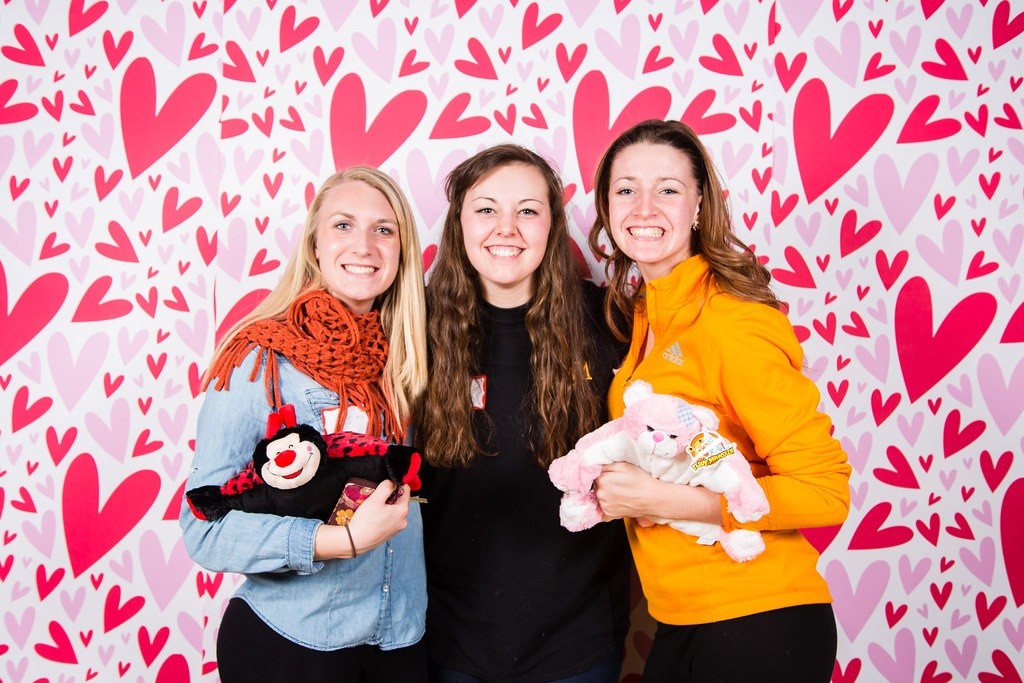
[345,524,357,558]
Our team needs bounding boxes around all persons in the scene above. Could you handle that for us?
[180,167,428,681]
[588,118,852,682]
[409,145,635,682]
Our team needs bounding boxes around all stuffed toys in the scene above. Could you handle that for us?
[186,404,422,527]
[549,380,771,565]
